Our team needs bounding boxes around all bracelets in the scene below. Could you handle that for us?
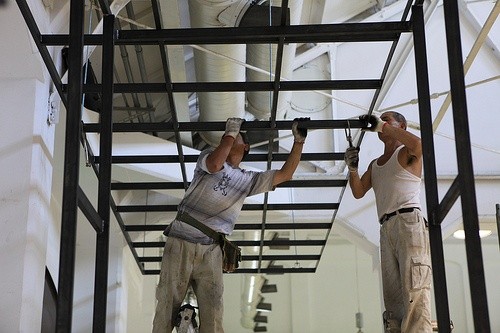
[350,170,357,172]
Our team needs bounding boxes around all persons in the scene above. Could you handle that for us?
[343,112,432,333]
[152,117,310,333]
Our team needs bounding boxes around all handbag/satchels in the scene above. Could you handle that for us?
[220,235,241,273]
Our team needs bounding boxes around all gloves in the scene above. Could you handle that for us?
[292,117,310,144]
[358,114,387,134]
[223,118,244,141]
[345,147,360,173]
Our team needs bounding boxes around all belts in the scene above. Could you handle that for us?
[380,208,414,225]
